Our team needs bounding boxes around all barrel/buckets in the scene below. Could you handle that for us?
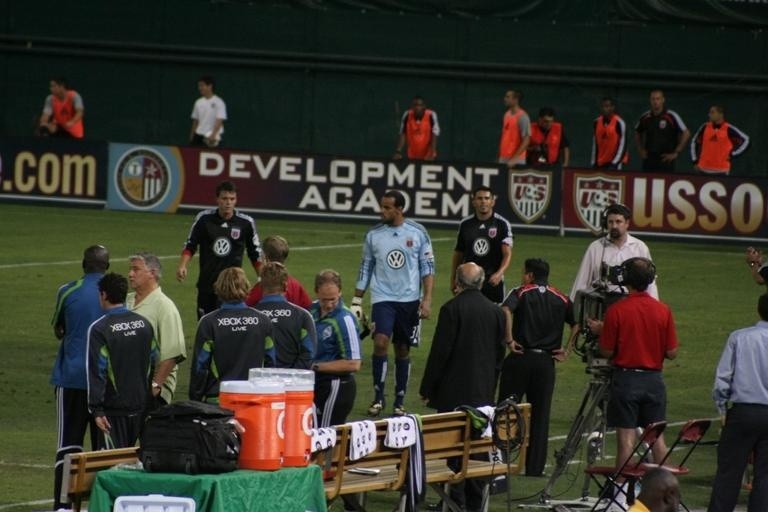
[248,368,315,468]
[219,381,286,471]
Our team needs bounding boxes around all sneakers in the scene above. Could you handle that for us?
[393,403,404,416]
[367,400,385,415]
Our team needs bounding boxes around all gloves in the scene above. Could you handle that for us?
[349,297,363,321]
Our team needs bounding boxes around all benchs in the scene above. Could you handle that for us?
[57,401,533,512]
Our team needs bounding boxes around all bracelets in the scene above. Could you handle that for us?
[397,151,404,154]
[507,339,513,346]
[673,150,681,156]
[749,260,756,267]
[351,297,363,306]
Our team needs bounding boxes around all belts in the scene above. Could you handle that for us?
[521,348,546,353]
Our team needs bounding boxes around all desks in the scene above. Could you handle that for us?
[88,463,328,512]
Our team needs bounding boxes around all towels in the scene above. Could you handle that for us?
[343,420,378,461]
[310,428,337,453]
[381,416,415,448]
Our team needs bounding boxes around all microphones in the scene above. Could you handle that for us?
[619,285,627,293]
[601,226,619,239]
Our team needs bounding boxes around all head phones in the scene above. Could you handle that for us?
[616,257,655,286]
[600,204,631,229]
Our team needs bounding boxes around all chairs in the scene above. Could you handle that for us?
[583,420,669,512]
[622,418,713,511]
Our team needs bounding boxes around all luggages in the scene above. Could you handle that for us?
[136,401,240,475]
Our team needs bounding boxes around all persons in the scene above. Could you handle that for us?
[54,244,187,511]
[188,74,227,149]
[177,184,360,428]
[625,468,679,512]
[39,76,84,138]
[420,262,505,511]
[590,97,627,171]
[746,246,768,285]
[394,96,441,162]
[635,89,690,174]
[527,105,571,168]
[449,187,514,307]
[500,259,579,477]
[570,205,660,303]
[350,192,434,416]
[691,104,750,176]
[498,89,531,166]
[709,292,767,511]
[588,258,668,511]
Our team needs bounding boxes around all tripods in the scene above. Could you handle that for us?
[539,373,652,504]
